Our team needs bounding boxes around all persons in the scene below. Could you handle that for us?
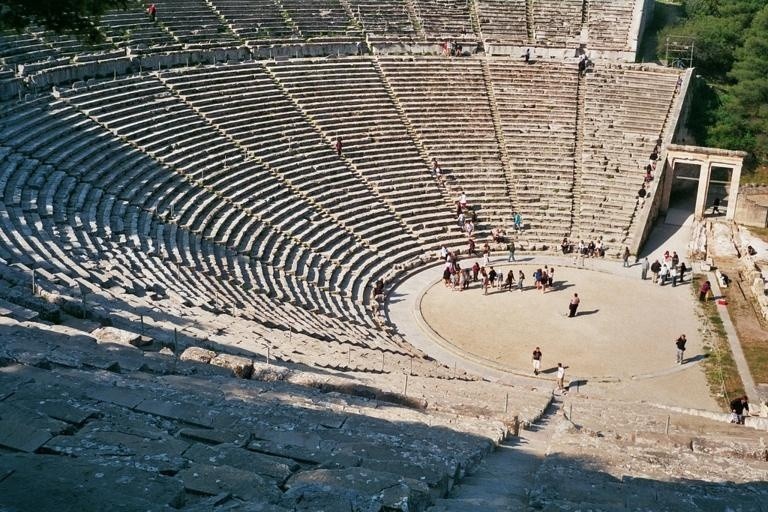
[655,136,662,153]
[747,246,756,256]
[711,195,722,214]
[440,38,463,57]
[674,334,687,365]
[730,395,751,424]
[335,138,343,157]
[531,346,543,375]
[556,362,565,394]
[434,165,444,185]
[148,2,157,23]
[374,277,384,295]
[439,191,687,318]
[699,281,710,302]
[430,158,439,179]
[375,293,387,305]
[677,51,687,69]
[676,75,682,93]
[637,185,646,208]
[646,163,652,182]
[649,150,657,169]
[521,48,531,62]
[578,58,586,79]
